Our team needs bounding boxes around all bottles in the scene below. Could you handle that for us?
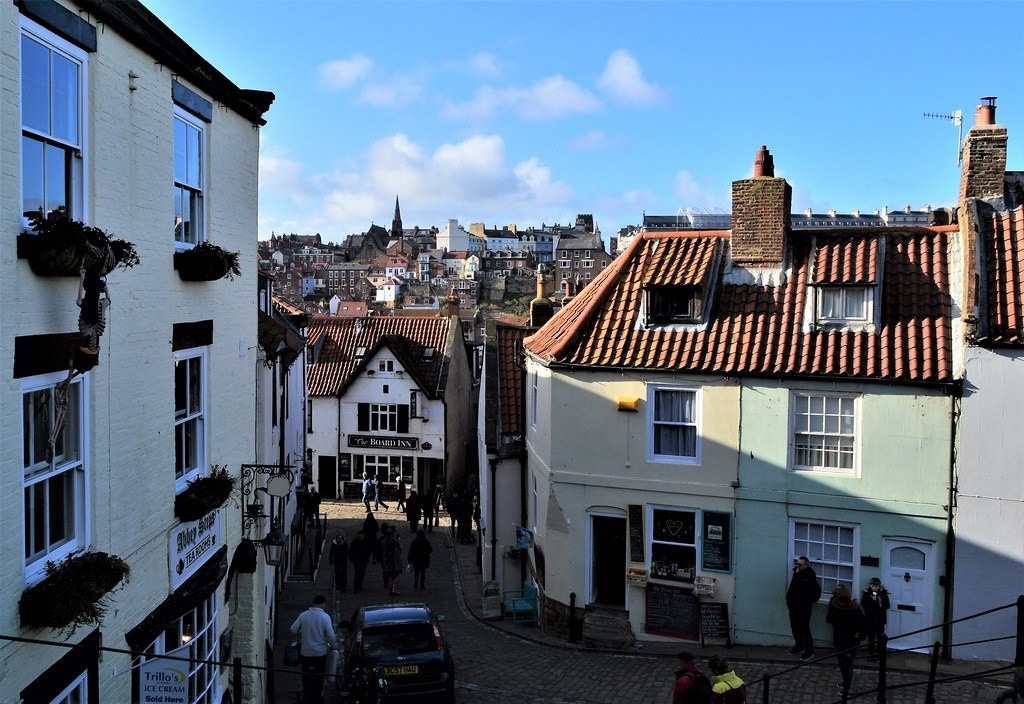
[652,558,694,579]
[628,568,647,582]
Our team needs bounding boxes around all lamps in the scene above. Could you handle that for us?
[249,515,287,567]
[396,371,404,375]
[367,370,375,375]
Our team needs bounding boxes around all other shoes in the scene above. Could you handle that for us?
[373,507,378,511]
[400,511,405,513]
[395,508,399,511]
[386,506,389,511]
[413,583,418,589]
[420,586,425,590]
[365,509,371,513]
[391,588,400,594]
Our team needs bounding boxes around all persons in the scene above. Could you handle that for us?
[302,473,473,594]
[704,655,747,704]
[860,577,890,663]
[289,594,336,703]
[785,556,815,662]
[671,650,714,704]
[825,585,867,698]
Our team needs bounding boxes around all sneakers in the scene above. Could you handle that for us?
[838,691,849,698]
[788,646,805,655]
[837,682,844,689]
[800,651,814,660]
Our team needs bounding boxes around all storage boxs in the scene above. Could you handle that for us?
[627,566,649,587]
[694,575,717,597]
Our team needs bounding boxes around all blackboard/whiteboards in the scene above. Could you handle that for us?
[701,509,732,574]
[699,602,730,637]
[627,503,647,565]
[645,581,701,641]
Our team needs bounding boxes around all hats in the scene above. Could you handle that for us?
[373,475,378,478]
[417,529,425,536]
[677,650,694,662]
[396,476,401,481]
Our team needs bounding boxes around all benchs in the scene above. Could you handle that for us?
[502,580,538,629]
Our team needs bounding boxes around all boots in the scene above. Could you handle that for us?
[428,526,433,532]
[423,524,427,530]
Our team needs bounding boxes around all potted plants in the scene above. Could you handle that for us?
[15,210,141,278]
[174,463,246,523]
[15,545,133,643]
[174,239,243,283]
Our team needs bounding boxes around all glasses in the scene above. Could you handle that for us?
[796,562,806,567]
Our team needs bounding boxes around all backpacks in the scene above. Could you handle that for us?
[682,670,713,704]
[830,613,867,658]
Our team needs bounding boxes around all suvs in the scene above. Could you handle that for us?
[334,603,455,704]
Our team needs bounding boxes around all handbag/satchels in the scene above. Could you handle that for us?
[324,649,339,683]
[283,639,300,666]
